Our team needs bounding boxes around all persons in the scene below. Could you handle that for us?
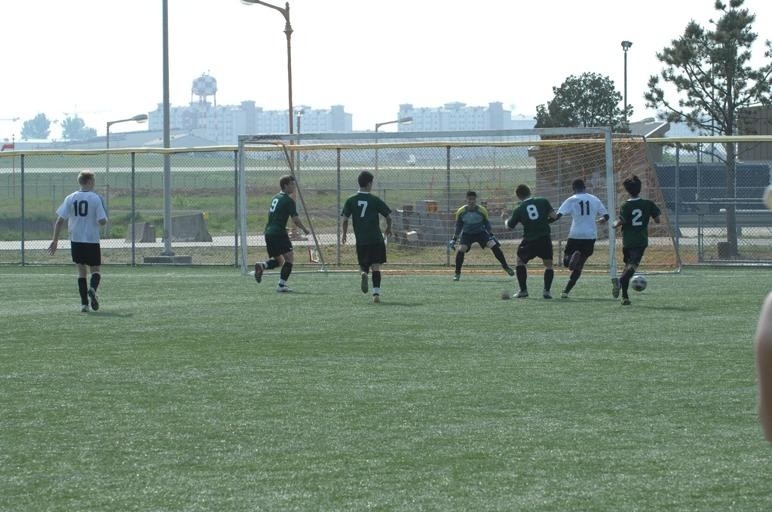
[501,184,557,299]
[48,171,108,313]
[341,171,393,303]
[450,191,515,281]
[548,179,609,298]
[255,175,309,292]
[612,174,661,306]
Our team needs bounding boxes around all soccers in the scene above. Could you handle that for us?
[632,276,647,291]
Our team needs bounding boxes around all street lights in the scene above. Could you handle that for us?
[294,105,304,216]
[242,0,311,242]
[372,114,415,184]
[622,38,632,135]
[103,114,149,238]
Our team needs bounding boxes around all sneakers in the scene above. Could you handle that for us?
[513,290,568,300]
[453,275,460,280]
[360,271,369,294]
[88,287,99,310]
[504,266,514,277]
[81,305,88,311]
[611,278,631,306]
[568,250,582,271]
[276,283,293,292]
[373,294,380,303]
[254,262,264,283]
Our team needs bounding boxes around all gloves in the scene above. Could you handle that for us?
[449,239,457,251]
[489,232,495,240]
